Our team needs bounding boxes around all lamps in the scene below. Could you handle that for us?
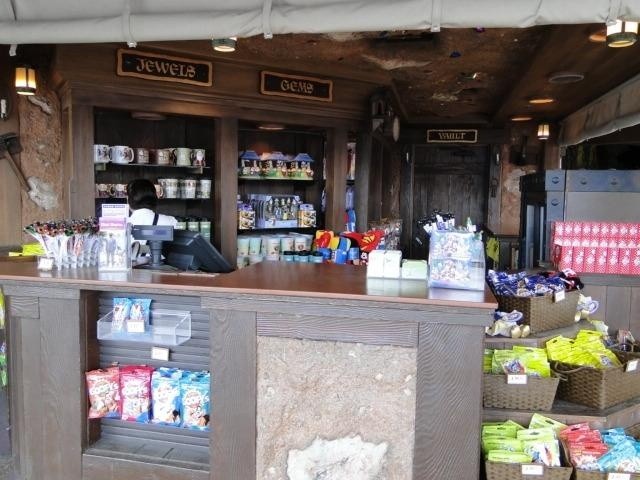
[212,36,237,52]
[536,122,551,141]
[606,19,639,48]
[15,64,37,96]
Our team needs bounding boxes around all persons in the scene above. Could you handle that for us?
[105,232,116,269]
[126,179,177,260]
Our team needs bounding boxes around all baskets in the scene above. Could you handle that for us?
[552,342,640,410]
[485,422,640,480]
[483,371,568,411]
[493,289,579,333]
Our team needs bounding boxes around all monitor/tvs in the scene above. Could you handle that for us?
[162,228,235,274]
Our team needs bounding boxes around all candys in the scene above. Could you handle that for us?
[20,216,100,255]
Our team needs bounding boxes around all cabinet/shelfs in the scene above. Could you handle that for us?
[49,42,385,270]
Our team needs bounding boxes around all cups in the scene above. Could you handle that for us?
[156,176,212,199]
[93,144,206,167]
[175,216,212,240]
[95,183,163,199]
[237,232,323,269]
